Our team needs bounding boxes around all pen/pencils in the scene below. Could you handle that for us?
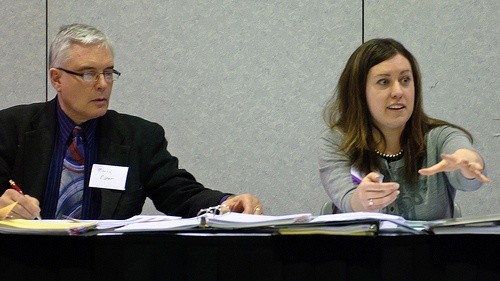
[9,180,42,220]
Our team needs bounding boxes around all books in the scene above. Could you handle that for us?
[0,211,500,236]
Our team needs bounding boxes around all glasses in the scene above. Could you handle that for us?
[55,68,121,83]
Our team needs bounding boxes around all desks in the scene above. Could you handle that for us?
[0,218,500,281]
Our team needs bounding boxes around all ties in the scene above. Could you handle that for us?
[54,126,85,220]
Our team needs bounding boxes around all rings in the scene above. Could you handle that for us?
[256,207,260,210]
[368,199,374,209]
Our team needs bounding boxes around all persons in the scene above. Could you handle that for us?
[0,22,265,221]
[319,38,492,220]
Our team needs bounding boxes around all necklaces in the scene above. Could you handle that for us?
[372,144,404,158]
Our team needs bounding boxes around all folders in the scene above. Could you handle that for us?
[277,220,381,237]
[430,212,499,234]
[114,223,275,233]
